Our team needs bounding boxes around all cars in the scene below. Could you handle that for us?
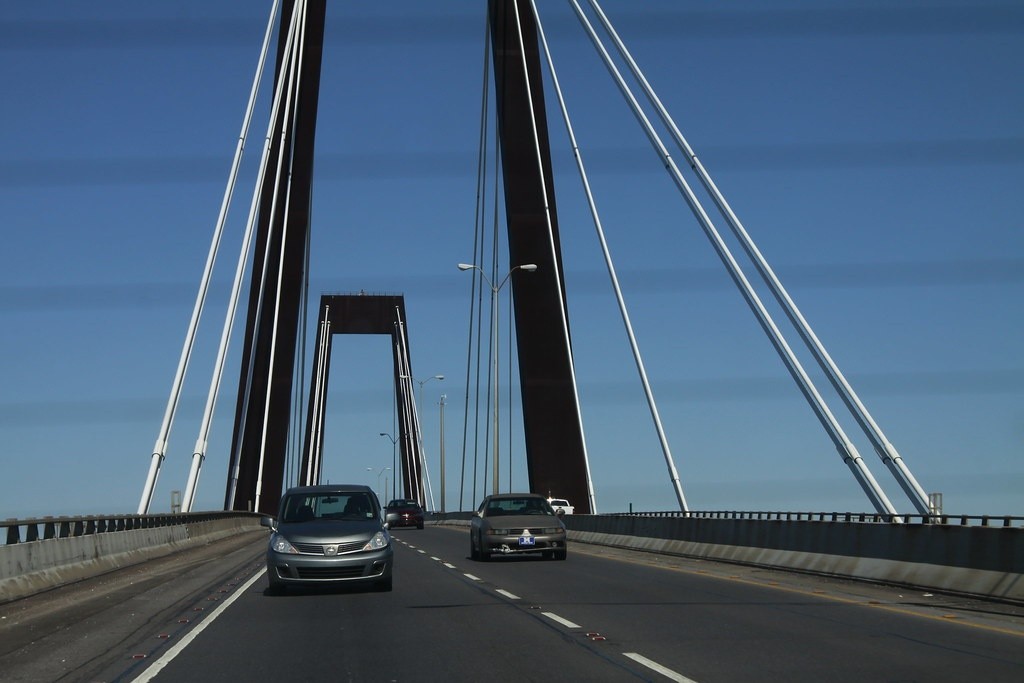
[381,499,425,530]
[261,484,399,591]
[469,493,568,560]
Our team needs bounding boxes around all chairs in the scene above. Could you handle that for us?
[488,508,505,516]
[296,505,313,522]
[344,496,368,516]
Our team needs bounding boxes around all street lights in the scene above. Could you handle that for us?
[399,374,443,496]
[459,263,539,494]
[381,433,409,500]
[365,467,390,499]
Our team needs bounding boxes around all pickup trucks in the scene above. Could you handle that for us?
[547,497,576,516]
